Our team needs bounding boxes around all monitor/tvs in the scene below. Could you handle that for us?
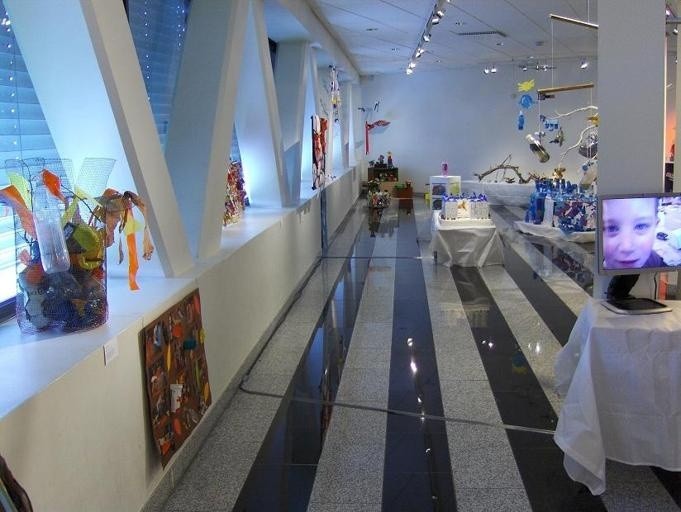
[597,192,681,315]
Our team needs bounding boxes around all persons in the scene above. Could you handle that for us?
[602,197,669,270]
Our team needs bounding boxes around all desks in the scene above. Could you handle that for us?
[581,297,680,474]
[431,209,496,269]
[429,176,462,209]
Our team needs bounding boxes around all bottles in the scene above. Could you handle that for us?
[31,181,71,274]
[517,111,525,130]
[525,134,550,164]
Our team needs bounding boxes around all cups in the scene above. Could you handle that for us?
[441,160,449,176]
